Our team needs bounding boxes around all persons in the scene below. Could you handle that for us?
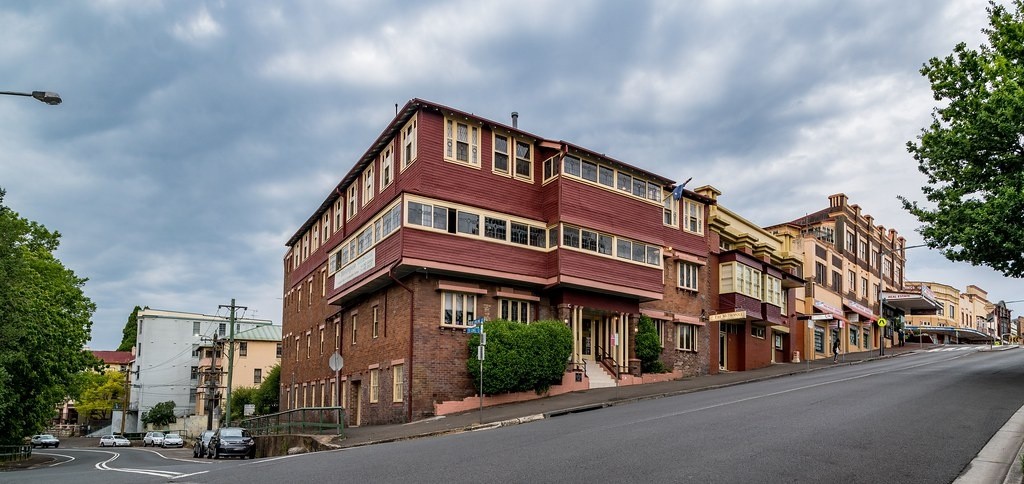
[898,329,905,347]
[833,337,841,363]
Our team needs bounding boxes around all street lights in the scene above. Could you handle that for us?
[873,241,931,357]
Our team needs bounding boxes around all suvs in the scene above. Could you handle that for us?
[100,434,131,446]
[30,434,60,449]
[207,426,256,460]
[142,431,164,447]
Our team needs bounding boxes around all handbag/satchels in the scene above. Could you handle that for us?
[835,347,840,354]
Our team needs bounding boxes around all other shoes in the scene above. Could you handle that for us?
[834,360,839,364]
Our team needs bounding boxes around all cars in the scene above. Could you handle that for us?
[191,430,216,458]
[162,433,183,448]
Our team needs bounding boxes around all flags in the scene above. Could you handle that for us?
[673,185,683,201]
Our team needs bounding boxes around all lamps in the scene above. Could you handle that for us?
[634,324,639,334]
[565,313,570,327]
